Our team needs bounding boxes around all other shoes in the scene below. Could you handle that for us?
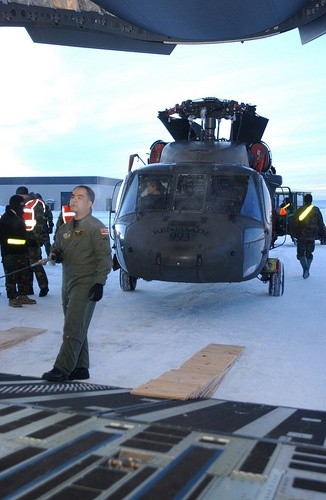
[7,296,22,308]
[27,289,34,295]
[19,295,37,305]
[39,287,49,297]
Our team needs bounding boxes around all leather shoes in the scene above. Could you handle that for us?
[40,365,70,382]
[68,364,90,379]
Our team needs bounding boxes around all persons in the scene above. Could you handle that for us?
[147,178,163,195]
[42,185,113,383]
[293,194,324,279]
[0,186,76,308]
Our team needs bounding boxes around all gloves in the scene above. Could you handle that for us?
[87,283,104,303]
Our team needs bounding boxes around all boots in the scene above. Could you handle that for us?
[300,257,313,278]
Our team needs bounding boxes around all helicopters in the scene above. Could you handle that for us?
[108,97,294,298]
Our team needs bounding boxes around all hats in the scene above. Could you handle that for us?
[303,194,313,203]
[15,186,28,194]
